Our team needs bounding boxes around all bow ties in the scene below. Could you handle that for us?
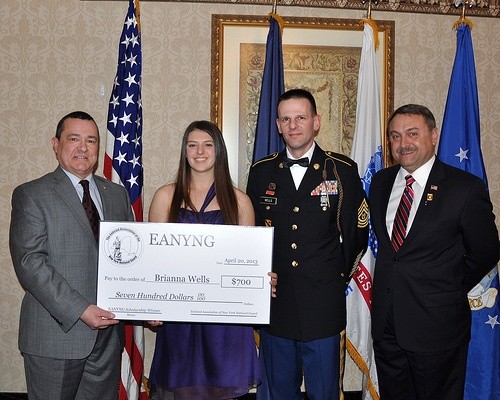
[282,155,310,169]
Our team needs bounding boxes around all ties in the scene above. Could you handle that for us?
[391,174,416,252]
[79,180,101,247]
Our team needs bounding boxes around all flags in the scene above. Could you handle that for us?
[102,0,148,400]
[437,20,500,400]
[252,14,287,164]
[343,19,380,400]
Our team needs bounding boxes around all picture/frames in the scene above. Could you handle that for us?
[208,16,394,191]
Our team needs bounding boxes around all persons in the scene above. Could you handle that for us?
[9,111,162,400]
[148,120,278,400]
[366,103,500,400]
[245,89,370,400]
